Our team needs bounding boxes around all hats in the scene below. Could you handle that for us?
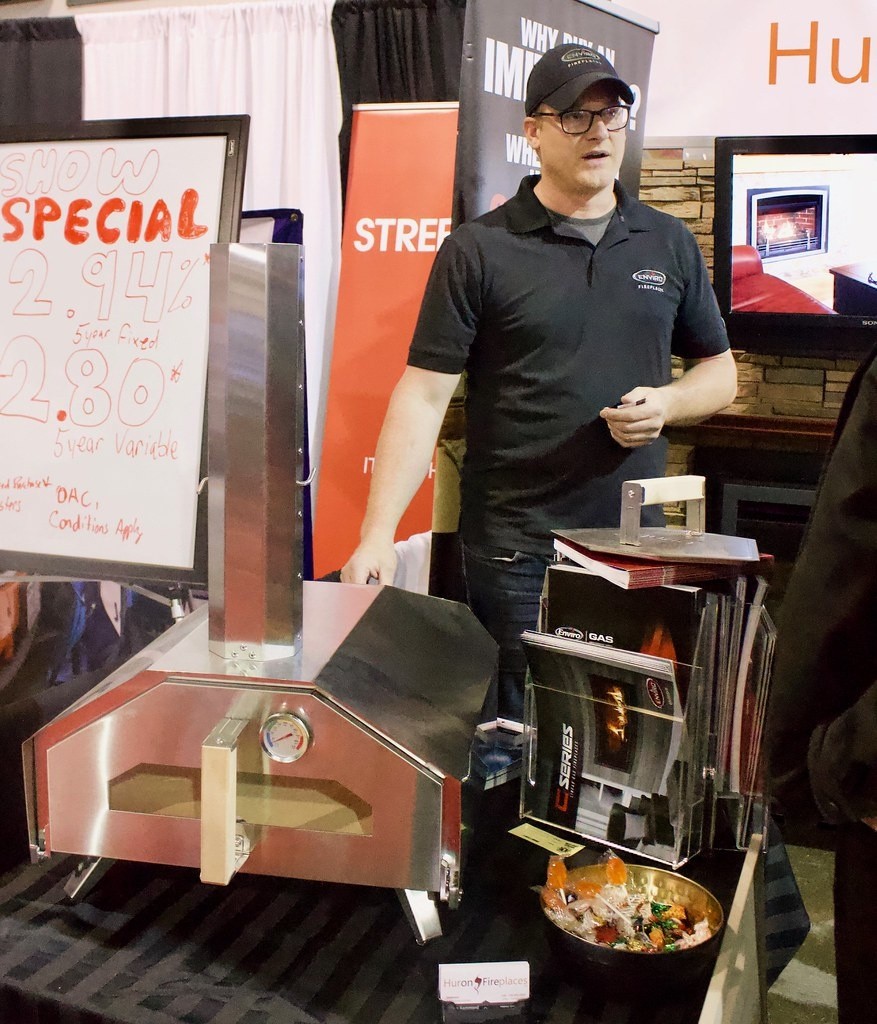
[526,43,634,116]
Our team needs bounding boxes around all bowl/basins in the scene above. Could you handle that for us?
[540,866,725,997]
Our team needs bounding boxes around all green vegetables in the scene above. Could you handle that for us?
[609,900,682,954]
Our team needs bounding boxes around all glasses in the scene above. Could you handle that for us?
[530,104,631,134]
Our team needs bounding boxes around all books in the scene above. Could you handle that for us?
[521,527,777,865]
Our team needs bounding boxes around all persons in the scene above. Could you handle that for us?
[340,44,739,722]
[754,350,877,1024]
